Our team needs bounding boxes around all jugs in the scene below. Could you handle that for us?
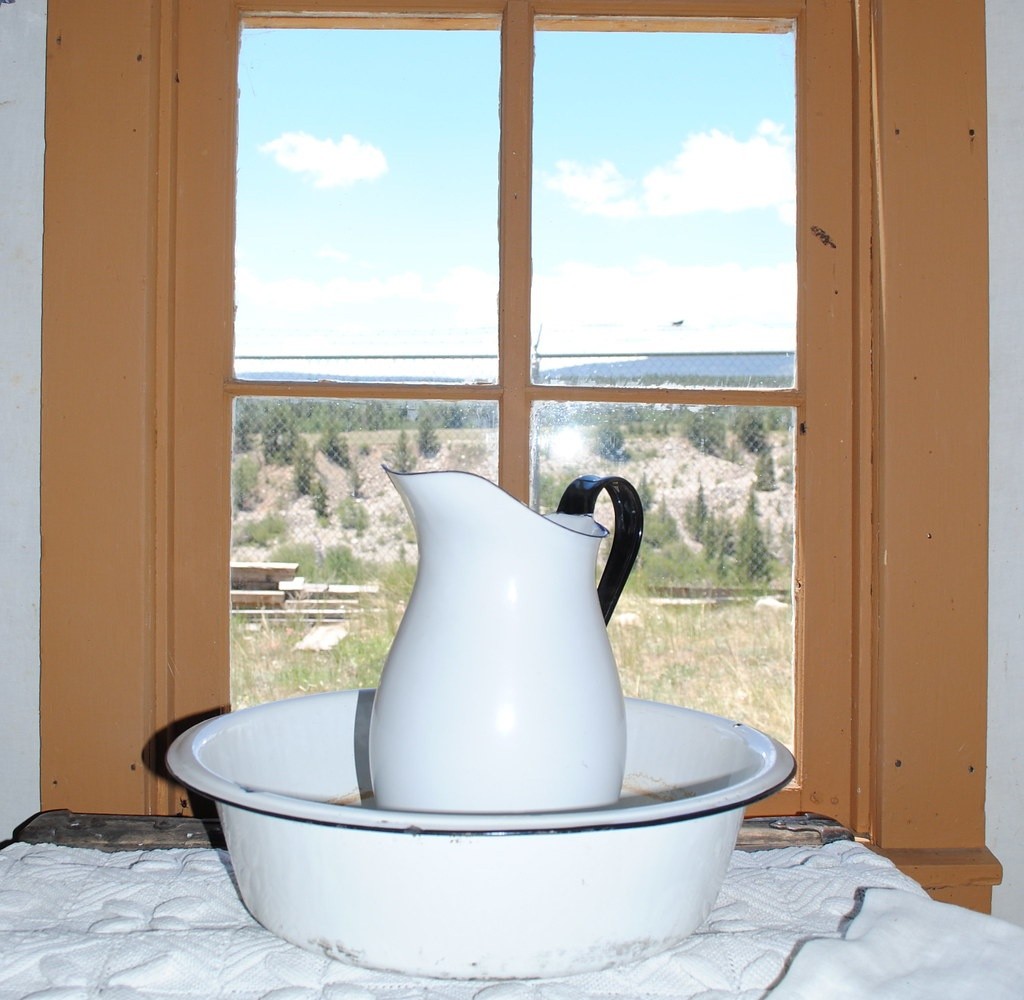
[369,466,646,816]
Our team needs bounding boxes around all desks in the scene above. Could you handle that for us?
[0,808,960,999]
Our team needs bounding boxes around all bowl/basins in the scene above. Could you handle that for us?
[164,682,796,980]
